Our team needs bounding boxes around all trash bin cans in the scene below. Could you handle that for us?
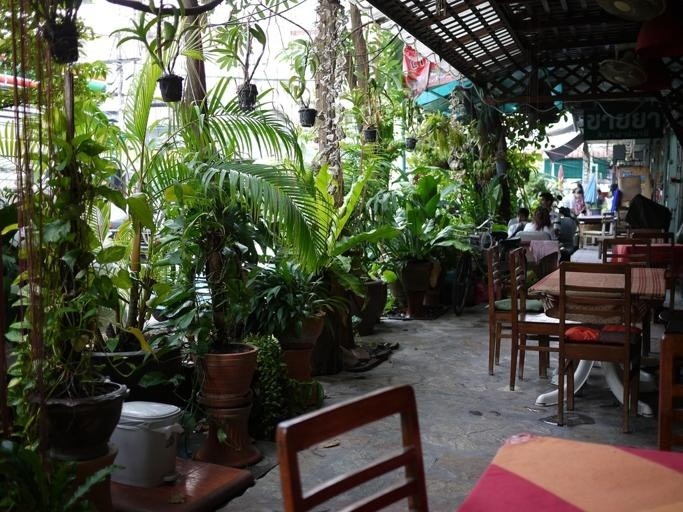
[107,402,183,488]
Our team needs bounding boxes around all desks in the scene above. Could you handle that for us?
[452,431,681,511]
[529,267,666,417]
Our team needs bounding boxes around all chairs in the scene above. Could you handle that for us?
[275,383,430,512]
[488,246,550,379]
[486,209,683,324]
[508,247,582,397]
[659,319,683,451]
[557,260,643,431]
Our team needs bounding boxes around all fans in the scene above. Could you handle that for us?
[594,0,666,23]
[597,50,648,89]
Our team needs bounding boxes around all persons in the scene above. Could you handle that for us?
[507,182,622,261]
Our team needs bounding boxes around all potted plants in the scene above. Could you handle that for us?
[1,2,265,512]
[250,0,485,424]
